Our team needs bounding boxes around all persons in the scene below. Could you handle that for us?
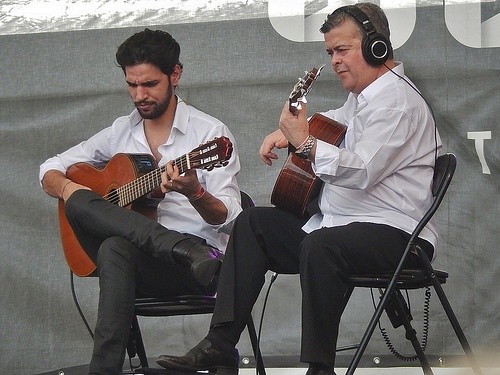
[156,3,443,375]
[39,28,244,375]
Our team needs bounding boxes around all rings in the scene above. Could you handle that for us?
[169,182,173,189]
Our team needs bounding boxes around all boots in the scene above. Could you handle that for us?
[173,238,225,287]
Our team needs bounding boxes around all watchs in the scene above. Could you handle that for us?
[295,135,315,159]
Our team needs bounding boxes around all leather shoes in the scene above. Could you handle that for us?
[156,336,239,375]
[304,364,337,375]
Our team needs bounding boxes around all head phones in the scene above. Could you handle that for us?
[332,5,390,67]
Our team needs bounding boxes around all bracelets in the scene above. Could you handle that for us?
[187,185,205,201]
[296,135,309,149]
[62,181,71,199]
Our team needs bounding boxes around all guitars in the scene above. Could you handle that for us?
[57,135,233,278]
[270,68,347,217]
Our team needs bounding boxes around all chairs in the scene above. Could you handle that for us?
[132,191,267,374]
[342,153,485,375]
